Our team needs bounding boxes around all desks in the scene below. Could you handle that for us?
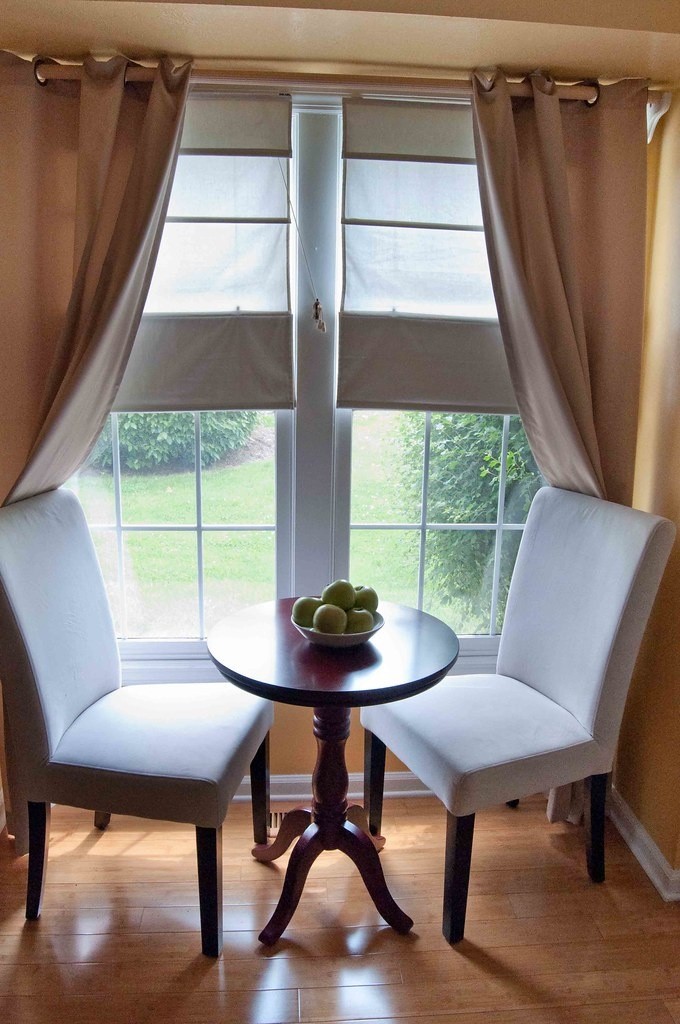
[208,599,460,945]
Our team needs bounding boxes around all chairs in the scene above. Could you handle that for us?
[360,486,677,944]
[0,490,276,960]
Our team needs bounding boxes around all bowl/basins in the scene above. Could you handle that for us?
[290,611,384,647]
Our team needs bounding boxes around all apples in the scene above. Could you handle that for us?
[292,579,378,634]
